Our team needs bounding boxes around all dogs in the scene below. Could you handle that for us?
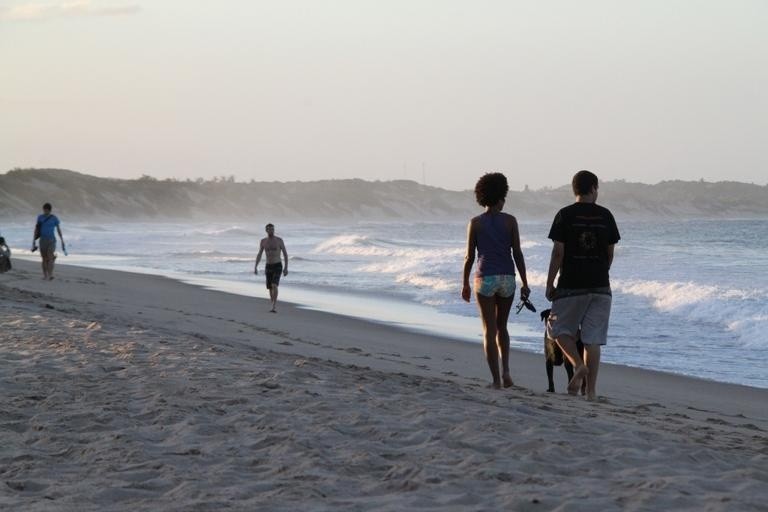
[539,308,584,393]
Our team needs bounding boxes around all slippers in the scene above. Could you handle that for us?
[515,294,536,314]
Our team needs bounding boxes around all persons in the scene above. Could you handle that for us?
[0,235,12,274]
[544,168,622,402]
[254,222,288,314]
[461,171,530,388]
[31,203,65,281]
